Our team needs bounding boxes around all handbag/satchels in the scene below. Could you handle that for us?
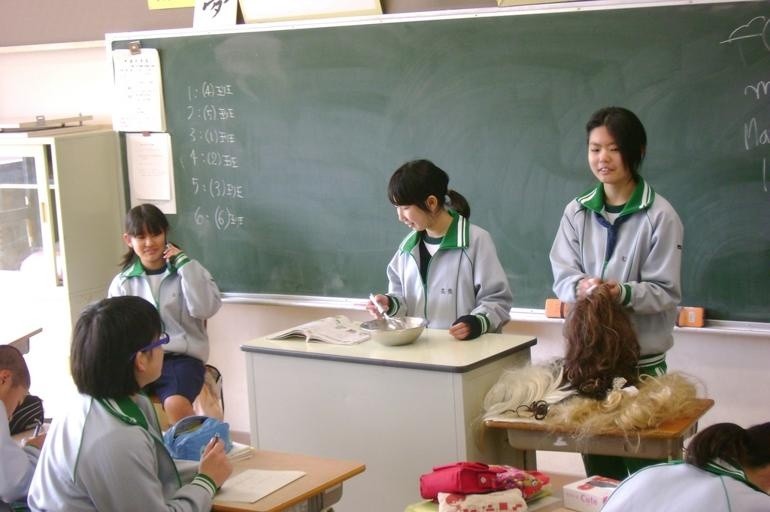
[419,463,507,500]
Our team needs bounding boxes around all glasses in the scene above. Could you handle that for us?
[129,333,169,362]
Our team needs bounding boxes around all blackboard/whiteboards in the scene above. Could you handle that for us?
[102,0,770,339]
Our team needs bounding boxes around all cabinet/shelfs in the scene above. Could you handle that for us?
[0,131,130,419]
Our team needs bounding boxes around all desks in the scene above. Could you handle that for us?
[191,449,367,512]
[483,390,714,473]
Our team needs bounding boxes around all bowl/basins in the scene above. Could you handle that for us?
[359,316,430,347]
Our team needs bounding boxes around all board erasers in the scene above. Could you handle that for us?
[676,306,705,328]
[544,299,574,318]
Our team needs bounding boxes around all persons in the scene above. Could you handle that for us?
[104,204,224,429]
[548,106,685,482]
[365,158,515,342]
[0,345,50,511]
[24,295,235,511]
[597,419,770,512]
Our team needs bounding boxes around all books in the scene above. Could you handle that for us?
[406,484,565,511]
[263,313,375,346]
[169,437,256,485]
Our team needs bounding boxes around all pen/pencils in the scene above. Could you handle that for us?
[215,433,219,443]
[33,422,41,437]
